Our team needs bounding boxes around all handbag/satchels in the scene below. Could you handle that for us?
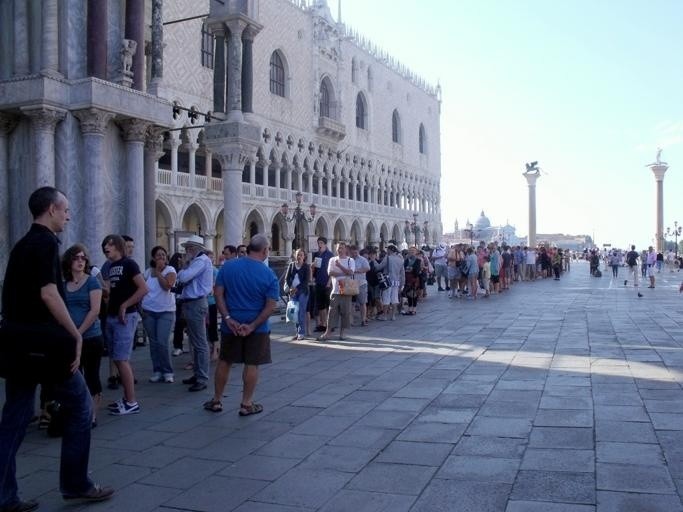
[376,271,392,290]
[285,295,299,323]
[283,262,294,294]
[338,272,360,296]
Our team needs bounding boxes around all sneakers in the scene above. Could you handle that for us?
[295,307,415,340]
[438,288,444,291]
[107,395,128,409]
[172,348,184,356]
[62,482,117,503]
[108,376,116,385]
[148,371,207,392]
[39,415,51,429]
[28,415,39,426]
[1,497,40,511]
[110,400,140,416]
[446,287,450,290]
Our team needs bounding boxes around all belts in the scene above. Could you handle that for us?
[182,296,203,303]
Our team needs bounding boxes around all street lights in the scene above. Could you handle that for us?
[498,229,510,244]
[281,191,317,249]
[405,212,429,250]
[464,224,483,246]
[667,221,682,258]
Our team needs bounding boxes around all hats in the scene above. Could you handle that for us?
[385,245,398,252]
[438,241,447,251]
[179,235,213,253]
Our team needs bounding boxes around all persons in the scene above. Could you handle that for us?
[432,241,571,301]
[572,245,683,292]
[25,233,279,437]
[284,237,435,341]
[1,187,115,507]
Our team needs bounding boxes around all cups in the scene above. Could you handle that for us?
[315,257,322,268]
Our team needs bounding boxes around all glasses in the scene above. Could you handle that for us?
[71,255,88,261]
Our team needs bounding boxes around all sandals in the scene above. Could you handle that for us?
[203,398,223,412]
[239,402,263,416]
